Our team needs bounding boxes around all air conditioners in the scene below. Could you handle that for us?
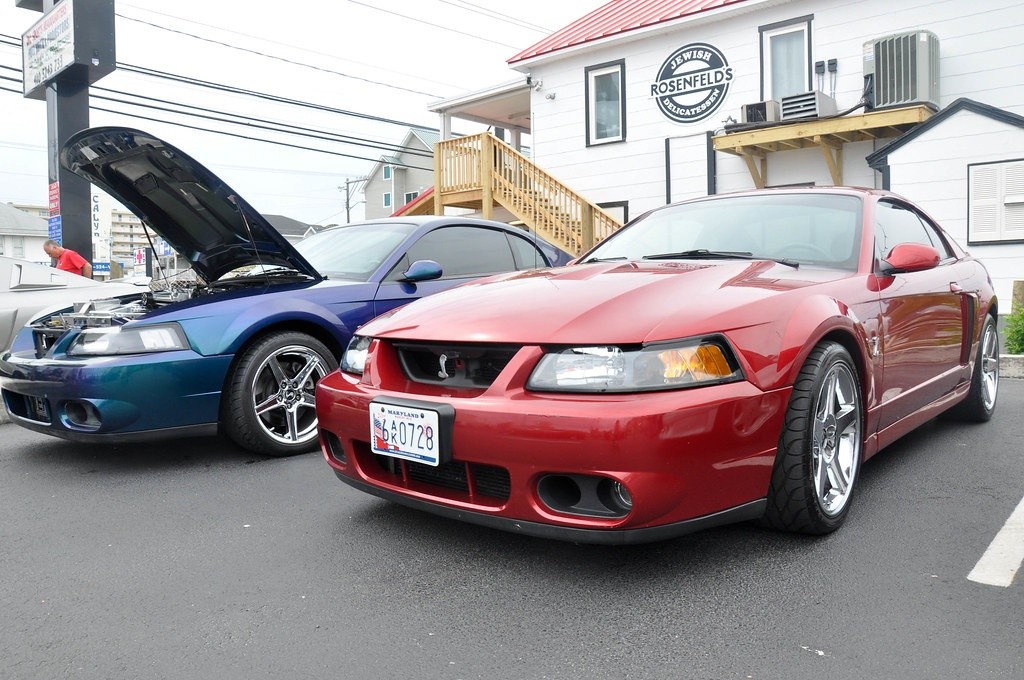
[780,91,838,121]
[741,100,780,122]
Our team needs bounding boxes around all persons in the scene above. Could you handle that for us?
[44,239,92,280]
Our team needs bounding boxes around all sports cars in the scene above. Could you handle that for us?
[0,255,155,358]
[314,184,1004,551]
[0,124,581,458]
